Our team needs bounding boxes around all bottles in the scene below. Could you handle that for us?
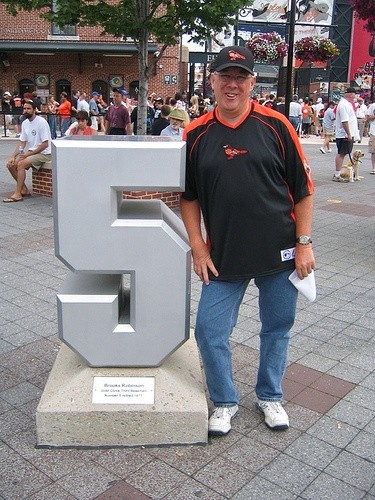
[18,145,23,154]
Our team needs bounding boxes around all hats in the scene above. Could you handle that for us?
[345,87,359,93]
[113,87,121,92]
[62,92,68,97]
[169,109,186,120]
[5,92,11,96]
[210,46,255,75]
[121,90,128,95]
[153,98,164,104]
[328,101,337,105]
[13,90,18,95]
[93,92,100,96]
[316,98,322,102]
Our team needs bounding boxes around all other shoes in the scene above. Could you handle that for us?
[320,148,325,154]
[332,174,349,183]
[327,148,331,152]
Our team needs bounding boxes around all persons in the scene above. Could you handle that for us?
[71,90,107,132]
[249,94,375,138]
[130,89,217,136]
[104,89,132,135]
[3,102,52,202]
[55,90,71,138]
[319,101,338,154]
[69,110,98,135]
[65,117,92,136]
[332,88,360,183]
[365,102,375,174]
[1,91,42,137]
[353,97,368,143]
[178,46,315,435]
[47,95,57,139]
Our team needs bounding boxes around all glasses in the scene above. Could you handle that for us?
[214,72,255,83]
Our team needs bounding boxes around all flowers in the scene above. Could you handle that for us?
[244,30,290,64]
[294,37,341,64]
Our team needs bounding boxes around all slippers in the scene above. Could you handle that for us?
[22,193,31,196]
[3,197,23,202]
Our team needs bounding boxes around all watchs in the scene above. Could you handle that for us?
[295,235,312,245]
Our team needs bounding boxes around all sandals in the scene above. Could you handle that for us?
[370,171,375,174]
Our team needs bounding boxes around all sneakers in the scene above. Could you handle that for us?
[256,399,289,429]
[208,404,240,434]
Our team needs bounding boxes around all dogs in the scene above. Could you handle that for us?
[340,150,365,181]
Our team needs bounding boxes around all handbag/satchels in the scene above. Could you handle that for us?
[318,109,325,118]
